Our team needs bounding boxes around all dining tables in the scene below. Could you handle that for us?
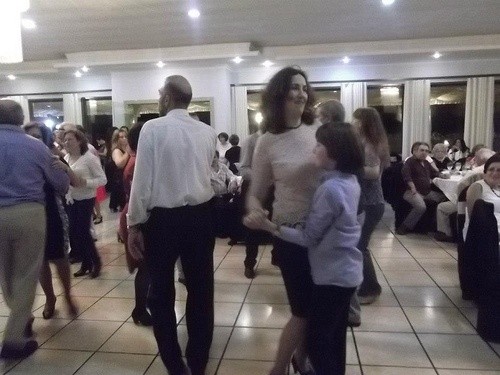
[432,162,475,202]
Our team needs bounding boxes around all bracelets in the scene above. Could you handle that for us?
[274,225,282,237]
[127,225,137,230]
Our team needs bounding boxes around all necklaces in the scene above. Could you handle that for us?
[490,184,500,198]
[289,123,306,130]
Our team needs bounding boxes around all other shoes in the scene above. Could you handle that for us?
[110,208,118,212]
[42,295,57,319]
[244,266,255,278]
[69,256,82,264]
[65,297,78,315]
[0,339,40,359]
[67,251,74,257]
[94,217,103,224]
[434,231,457,241]
[357,293,375,304]
[25,318,35,338]
[396,225,409,235]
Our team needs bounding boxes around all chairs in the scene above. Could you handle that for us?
[451,201,500,342]
[382,161,437,234]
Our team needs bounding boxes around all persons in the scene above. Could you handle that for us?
[0,67,390,375]
[396,137,500,344]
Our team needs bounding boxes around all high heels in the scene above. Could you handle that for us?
[73,264,93,276]
[131,306,154,327]
[290,354,314,375]
[91,262,100,278]
